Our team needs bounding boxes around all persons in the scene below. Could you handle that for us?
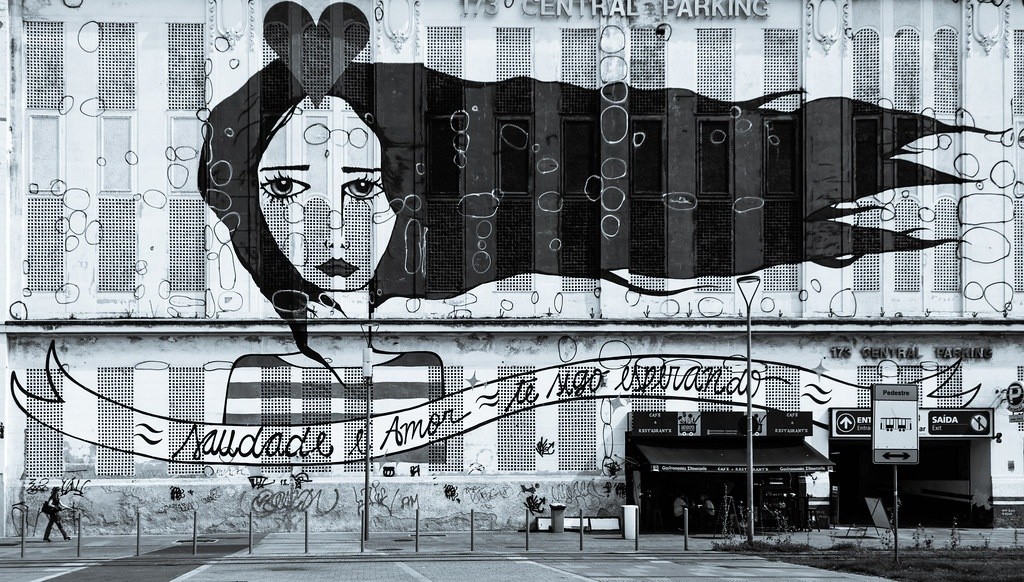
[700,494,715,531]
[674,492,690,532]
[43,486,71,542]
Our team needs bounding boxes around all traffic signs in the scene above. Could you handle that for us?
[828,407,871,439]
[873,383,920,465]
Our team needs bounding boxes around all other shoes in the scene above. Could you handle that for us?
[44,538,51,542]
[678,528,683,531]
[64,537,70,540]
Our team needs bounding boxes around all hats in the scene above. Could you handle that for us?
[52,487,60,490]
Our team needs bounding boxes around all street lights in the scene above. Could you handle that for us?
[736,274,762,546]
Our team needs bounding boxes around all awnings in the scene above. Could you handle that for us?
[634,441,836,473]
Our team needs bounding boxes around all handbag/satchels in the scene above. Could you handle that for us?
[42,501,57,515]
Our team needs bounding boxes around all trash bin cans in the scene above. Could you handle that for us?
[550,503,567,532]
[620,505,638,539]
